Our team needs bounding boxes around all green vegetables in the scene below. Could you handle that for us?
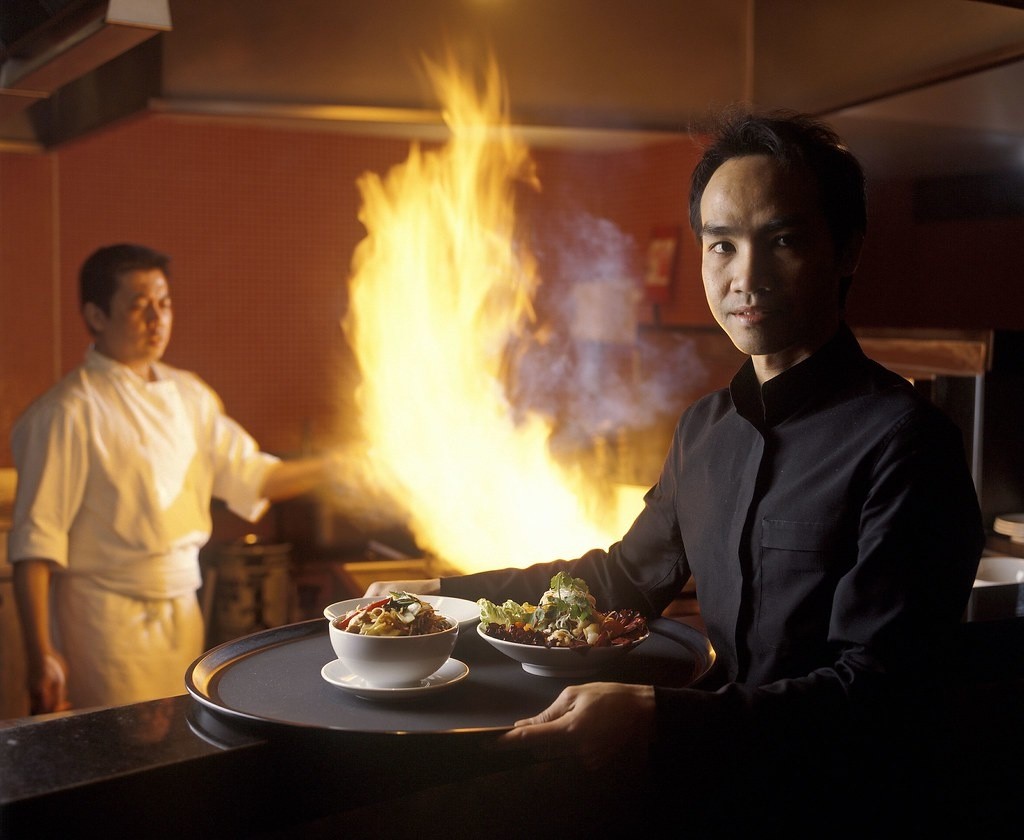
[477,567,594,630]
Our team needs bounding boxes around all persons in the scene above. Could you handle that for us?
[7,243,352,719]
[363,116,983,839]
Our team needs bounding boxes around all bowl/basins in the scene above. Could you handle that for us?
[323,595,481,640]
[328,614,459,688]
[476,622,650,678]
[972,556,1024,588]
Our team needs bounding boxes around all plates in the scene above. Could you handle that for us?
[320,659,469,701]
